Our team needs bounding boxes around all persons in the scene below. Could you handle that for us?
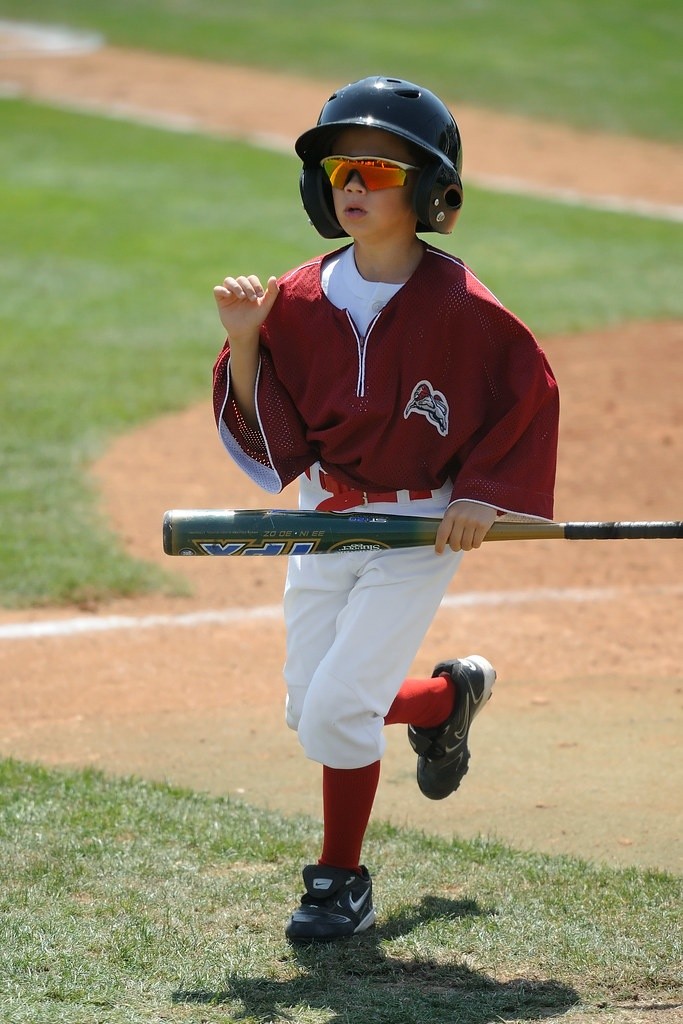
[210,74,561,945]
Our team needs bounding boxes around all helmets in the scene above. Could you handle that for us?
[295,76,463,239]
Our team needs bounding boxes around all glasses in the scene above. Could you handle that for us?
[319,155,428,190]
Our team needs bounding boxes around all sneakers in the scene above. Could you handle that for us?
[407,655,496,800]
[284,864,375,944]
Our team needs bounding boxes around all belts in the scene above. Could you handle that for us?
[305,468,433,512]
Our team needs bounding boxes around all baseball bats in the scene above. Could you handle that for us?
[160,500,682,559]
